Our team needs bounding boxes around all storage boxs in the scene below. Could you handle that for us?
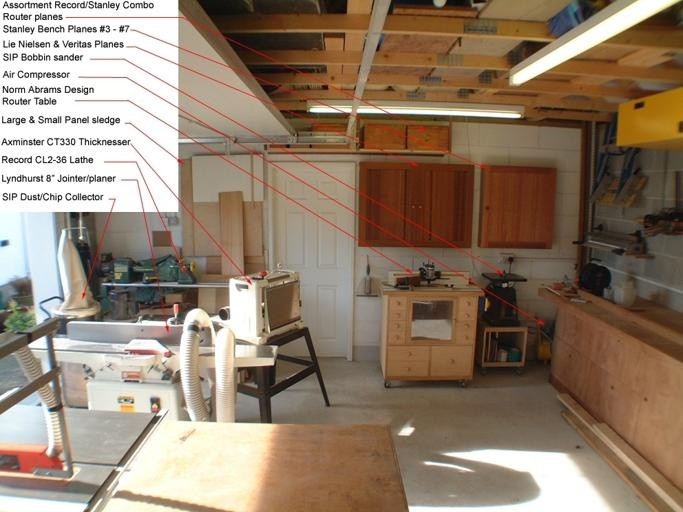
[310,124,447,152]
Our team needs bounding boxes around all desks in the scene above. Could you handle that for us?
[1,404,410,512]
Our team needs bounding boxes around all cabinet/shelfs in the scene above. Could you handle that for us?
[549,307,683,495]
[478,162,557,249]
[357,163,476,249]
[380,281,484,387]
